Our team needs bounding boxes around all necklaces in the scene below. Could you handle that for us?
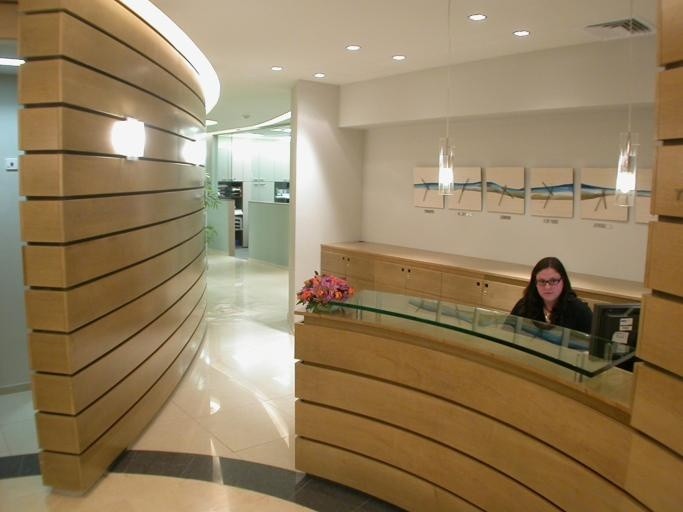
[543,304,552,314]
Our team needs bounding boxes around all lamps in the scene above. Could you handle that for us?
[616,0,635,207]
[111,118,145,160]
[437,0,454,196]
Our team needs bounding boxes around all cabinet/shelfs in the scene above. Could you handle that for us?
[217,134,290,183]
[575,291,641,313]
[446,273,527,309]
[377,260,442,301]
[322,253,375,293]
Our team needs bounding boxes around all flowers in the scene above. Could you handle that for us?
[296,272,356,310]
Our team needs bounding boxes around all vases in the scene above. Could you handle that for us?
[316,301,333,313]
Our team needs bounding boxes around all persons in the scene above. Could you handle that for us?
[501,257,593,353]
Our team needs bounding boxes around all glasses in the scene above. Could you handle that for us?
[535,278,562,285]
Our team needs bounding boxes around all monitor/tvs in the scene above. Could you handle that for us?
[591,302,640,367]
